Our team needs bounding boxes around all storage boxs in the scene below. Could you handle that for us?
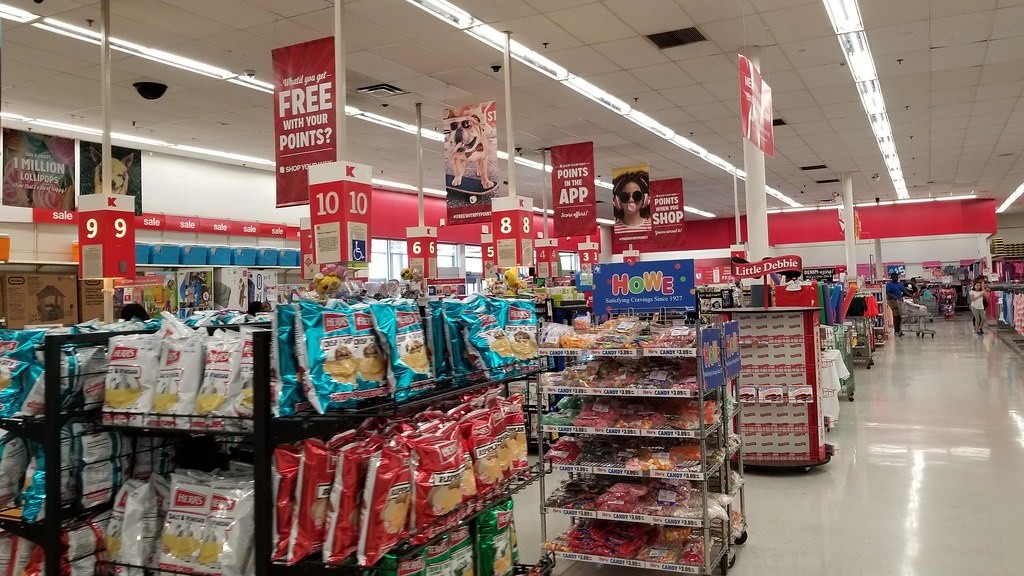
[277,248,297,266]
[143,271,177,318]
[278,284,310,305]
[248,269,278,310]
[3,274,78,330]
[207,245,231,264]
[149,242,180,264]
[215,268,248,312]
[256,247,277,266]
[177,268,214,319]
[135,241,148,263]
[77,276,105,324]
[231,246,256,265]
[113,271,145,322]
[180,243,207,264]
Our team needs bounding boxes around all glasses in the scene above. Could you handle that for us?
[620,191,643,203]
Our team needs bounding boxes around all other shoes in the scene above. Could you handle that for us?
[894,332,900,336]
[978,328,983,334]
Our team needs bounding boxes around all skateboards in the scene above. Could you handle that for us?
[445,174,499,205]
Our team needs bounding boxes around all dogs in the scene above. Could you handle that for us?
[449,114,495,189]
[89,145,135,195]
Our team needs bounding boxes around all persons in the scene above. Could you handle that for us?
[902,277,921,324]
[968,276,989,336]
[247,300,271,316]
[612,170,652,234]
[120,303,150,322]
[886,273,916,337]
[238,278,242,293]
[712,301,721,309]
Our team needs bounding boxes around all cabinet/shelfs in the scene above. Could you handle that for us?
[536,320,746,576]
[700,307,831,470]
[858,285,887,348]
[0,322,556,576]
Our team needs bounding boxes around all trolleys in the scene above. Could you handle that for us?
[898,296,936,339]
